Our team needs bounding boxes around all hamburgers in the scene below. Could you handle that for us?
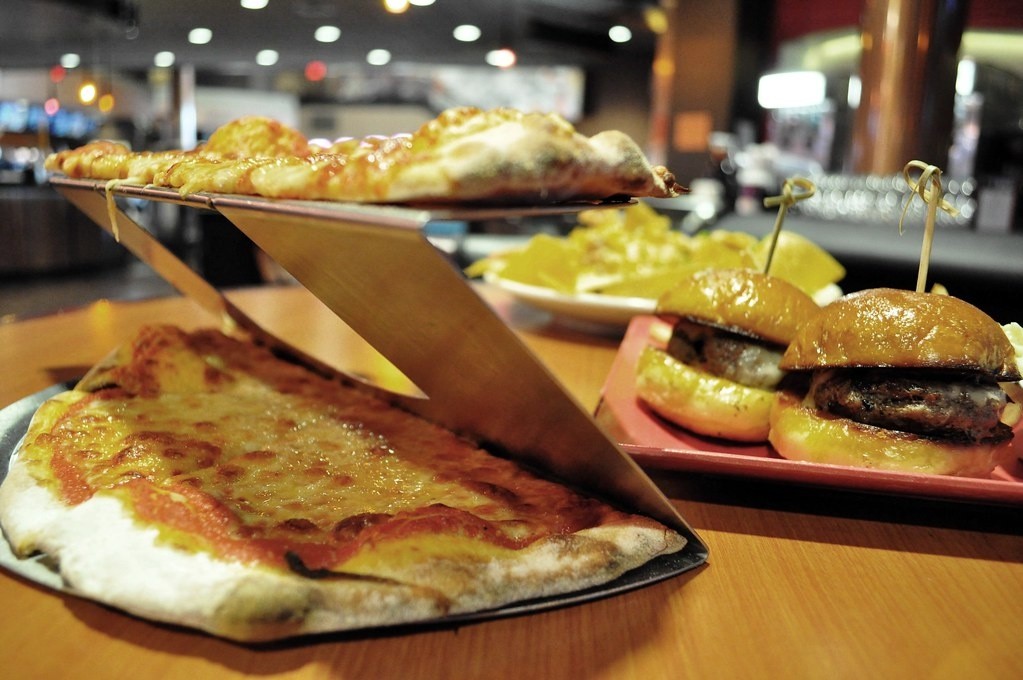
[636,263,1023,487]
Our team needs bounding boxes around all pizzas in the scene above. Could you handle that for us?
[0,104,691,645]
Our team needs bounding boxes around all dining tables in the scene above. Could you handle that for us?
[0,285,1023,680]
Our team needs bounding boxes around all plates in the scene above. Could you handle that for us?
[483,262,844,338]
[592,310,1023,503]
